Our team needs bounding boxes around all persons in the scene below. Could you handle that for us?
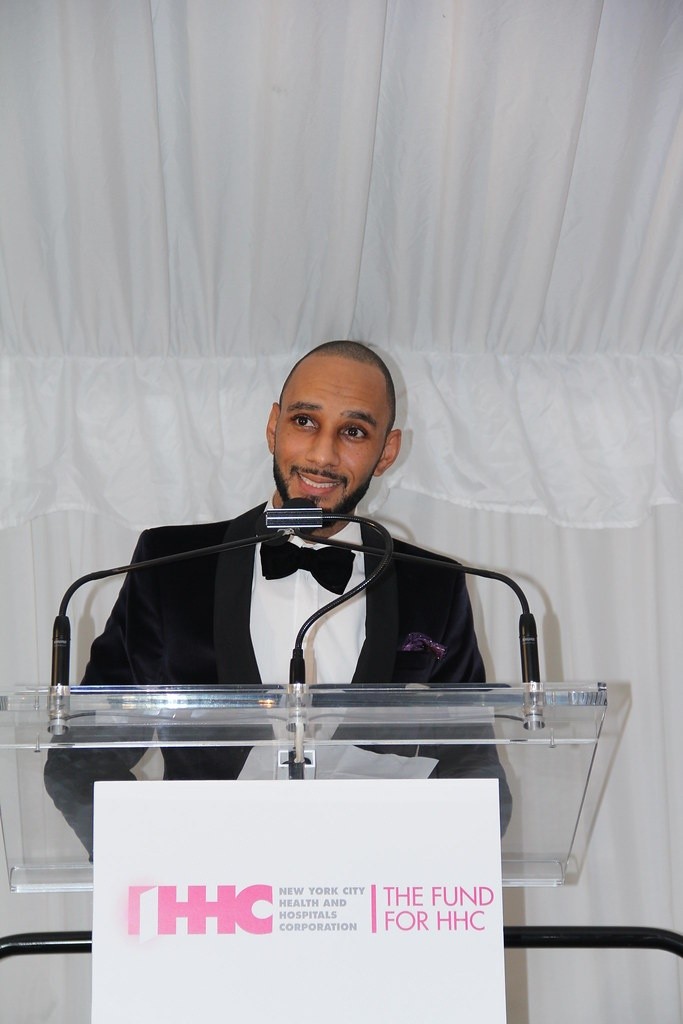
[42,340,513,864]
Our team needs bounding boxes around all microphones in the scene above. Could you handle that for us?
[50,494,541,692]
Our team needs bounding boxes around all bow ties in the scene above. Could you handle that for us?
[260,535,356,595]
